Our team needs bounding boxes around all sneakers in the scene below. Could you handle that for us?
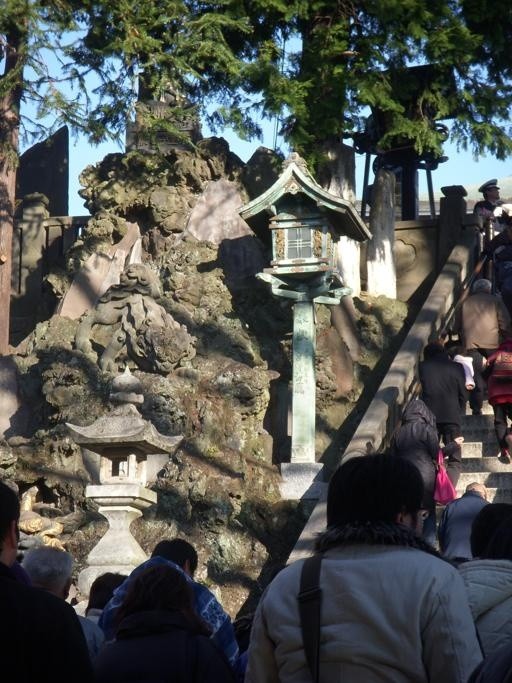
[472,408,484,415]
[505,433,512,445]
[501,448,511,464]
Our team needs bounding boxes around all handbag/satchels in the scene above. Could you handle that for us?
[433,464,457,506]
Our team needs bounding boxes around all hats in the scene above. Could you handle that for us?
[479,179,500,192]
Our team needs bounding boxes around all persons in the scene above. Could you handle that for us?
[479,334,512,465]
[382,398,466,553]
[240,447,487,682]
[0,480,91,682]
[477,202,511,316]
[453,276,512,416]
[453,500,511,683]
[451,345,476,393]
[414,340,472,487]
[83,570,129,642]
[438,480,491,570]
[94,536,241,674]
[19,545,107,666]
[89,555,239,682]
[471,177,506,215]
